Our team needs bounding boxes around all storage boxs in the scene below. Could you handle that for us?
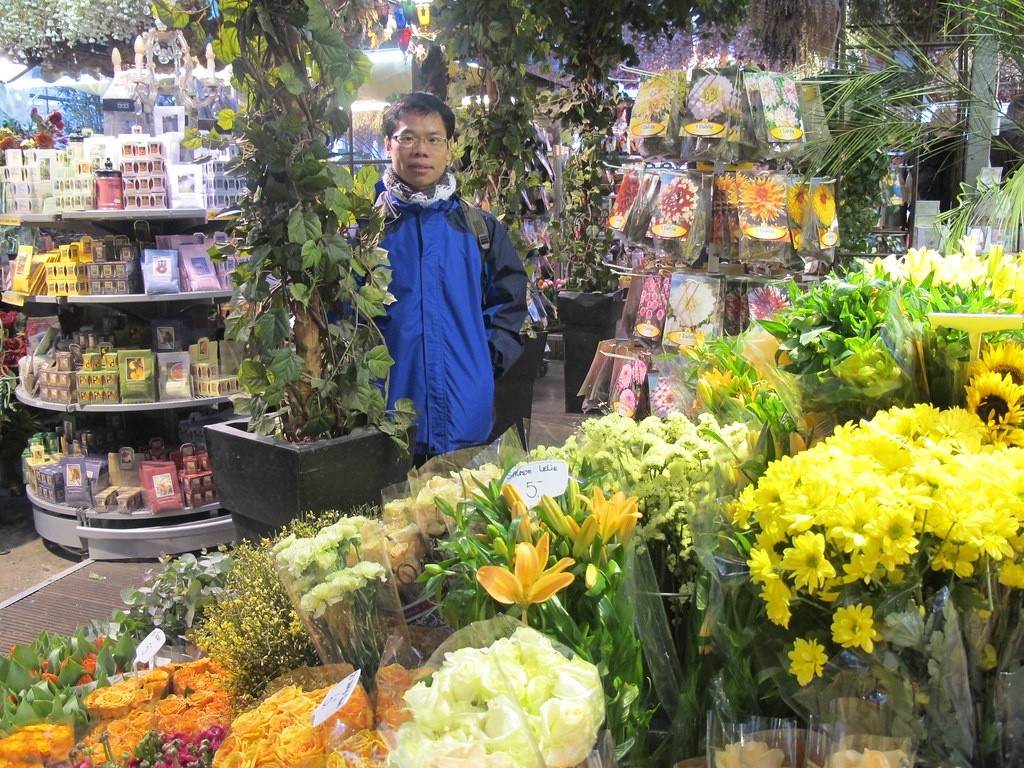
[0,106,246,514]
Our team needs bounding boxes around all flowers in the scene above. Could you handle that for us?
[666,246,1024,424]
[0,107,67,165]
[2,414,1023,768]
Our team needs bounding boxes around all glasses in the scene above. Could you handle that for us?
[390,135,448,148]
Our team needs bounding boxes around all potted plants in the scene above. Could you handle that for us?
[152,0,418,546]
[431,1,748,414]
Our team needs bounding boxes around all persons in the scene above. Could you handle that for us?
[917,96,1024,224]
[539,246,554,273]
[329,91,533,469]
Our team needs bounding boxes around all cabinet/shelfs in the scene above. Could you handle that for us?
[0,209,250,561]
